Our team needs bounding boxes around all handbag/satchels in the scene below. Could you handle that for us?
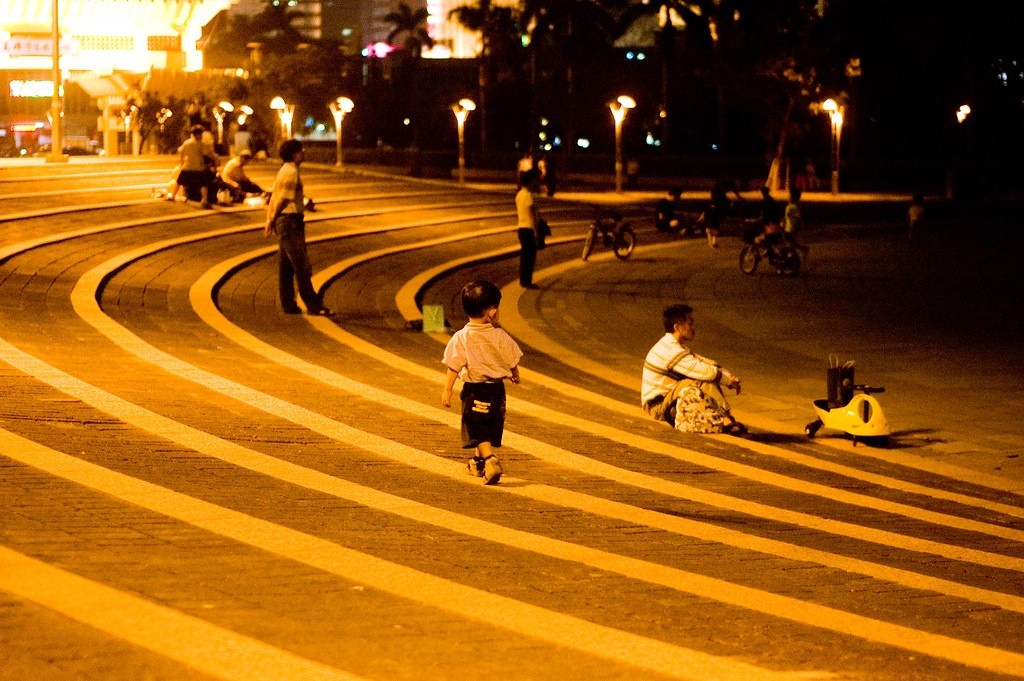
[674,386,724,435]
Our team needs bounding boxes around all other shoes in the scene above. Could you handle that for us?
[466,456,484,477]
[722,415,748,435]
[484,459,503,486]
[284,306,303,315]
[309,307,335,317]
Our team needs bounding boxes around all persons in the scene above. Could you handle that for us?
[441,280,523,484]
[904,195,926,242]
[265,140,337,316]
[641,303,748,435]
[163,124,270,208]
[697,173,810,265]
[514,171,548,288]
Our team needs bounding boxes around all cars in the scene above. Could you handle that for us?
[63,146,99,156]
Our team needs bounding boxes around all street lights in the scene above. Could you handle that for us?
[822,98,839,192]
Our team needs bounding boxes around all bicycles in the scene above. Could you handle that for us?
[582,203,636,260]
[739,221,801,278]
